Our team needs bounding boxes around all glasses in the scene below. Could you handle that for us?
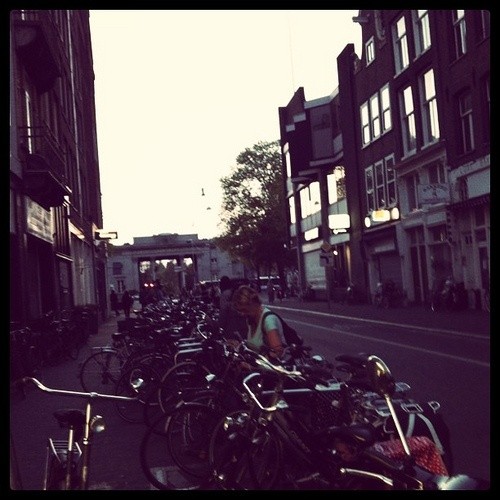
[235,309,250,317]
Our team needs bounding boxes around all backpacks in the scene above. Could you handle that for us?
[259,311,305,358]
[369,434,450,486]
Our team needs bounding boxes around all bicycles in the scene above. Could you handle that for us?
[1,277,478,492]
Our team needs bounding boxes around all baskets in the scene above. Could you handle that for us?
[225,425,266,460]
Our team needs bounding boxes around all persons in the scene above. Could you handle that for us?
[139,280,164,310]
[192,276,233,313]
[110,290,119,315]
[121,291,133,317]
[232,285,288,359]
[267,286,284,305]
